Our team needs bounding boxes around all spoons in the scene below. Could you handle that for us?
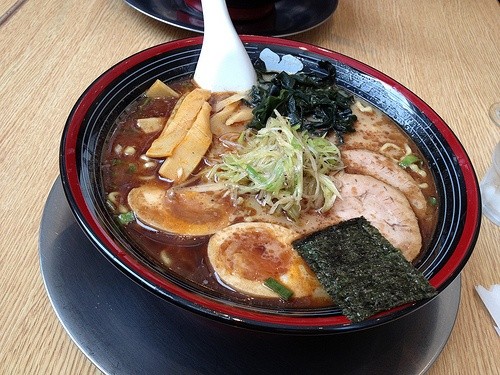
[192,0,258,94]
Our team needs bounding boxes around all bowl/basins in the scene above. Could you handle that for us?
[124,0,340,38]
[58,35,482,338]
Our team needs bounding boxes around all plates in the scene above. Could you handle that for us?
[37,171,461,375]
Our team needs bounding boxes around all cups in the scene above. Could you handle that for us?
[480,102,499,227]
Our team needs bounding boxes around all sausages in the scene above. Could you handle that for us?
[127,148,426,299]
